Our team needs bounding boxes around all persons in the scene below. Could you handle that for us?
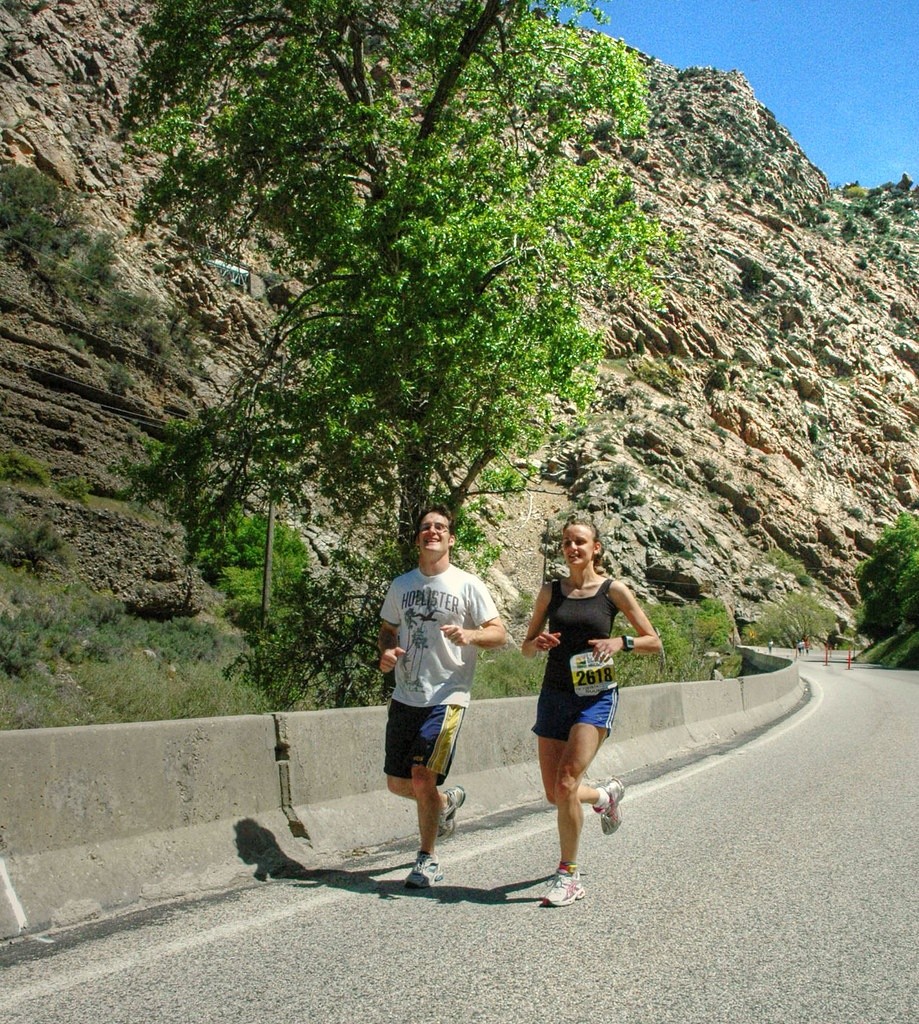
[768,637,774,654]
[521,518,663,909]
[376,503,510,891]
[799,638,810,656]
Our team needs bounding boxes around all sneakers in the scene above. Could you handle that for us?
[435,786,466,841]
[543,867,585,906]
[592,779,625,835]
[404,850,444,887]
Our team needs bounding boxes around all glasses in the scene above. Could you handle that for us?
[420,523,450,533]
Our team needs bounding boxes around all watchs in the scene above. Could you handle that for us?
[621,635,635,652]
